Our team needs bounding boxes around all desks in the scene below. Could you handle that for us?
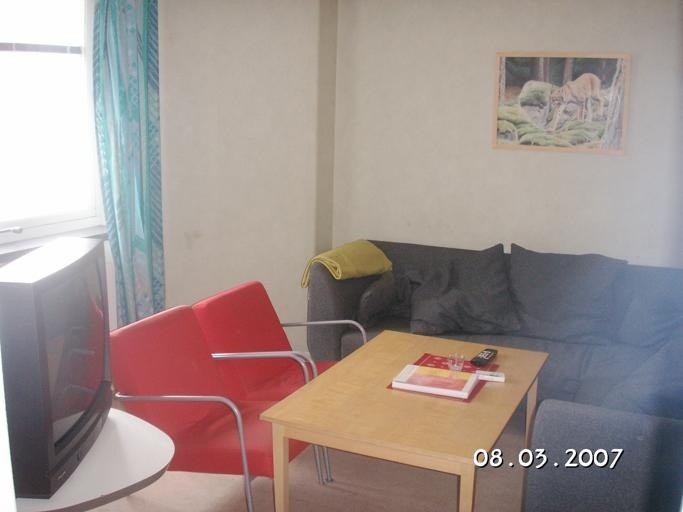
[15,408,176,512]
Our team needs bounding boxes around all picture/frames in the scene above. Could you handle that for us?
[490,51,631,156]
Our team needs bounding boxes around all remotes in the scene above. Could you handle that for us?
[470,348,498,367]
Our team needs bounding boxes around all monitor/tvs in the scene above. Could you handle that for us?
[0,234,114,499]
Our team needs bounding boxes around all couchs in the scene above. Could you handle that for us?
[307,240,683,512]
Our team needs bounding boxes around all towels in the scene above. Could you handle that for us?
[300,239,393,289]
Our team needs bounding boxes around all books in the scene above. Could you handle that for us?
[391,364,479,400]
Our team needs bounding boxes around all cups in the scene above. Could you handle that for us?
[448,352,464,371]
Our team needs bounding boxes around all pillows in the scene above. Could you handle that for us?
[510,243,628,346]
[410,244,522,335]
[604,264,683,349]
[601,338,683,420]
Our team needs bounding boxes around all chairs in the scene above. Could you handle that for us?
[192,281,368,482]
[109,304,324,512]
